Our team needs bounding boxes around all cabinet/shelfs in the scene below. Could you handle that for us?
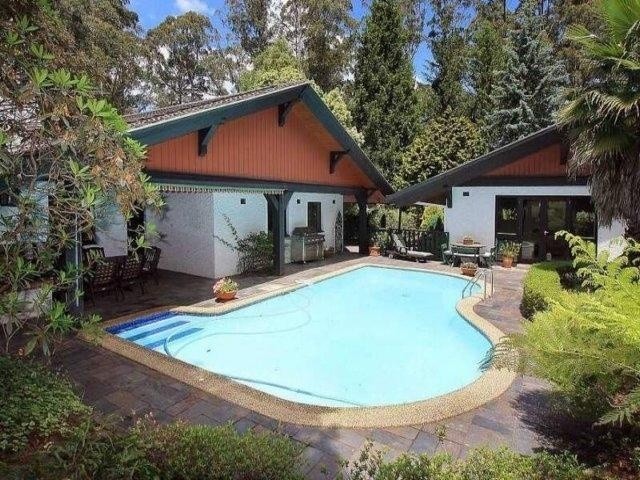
[290,234,325,266]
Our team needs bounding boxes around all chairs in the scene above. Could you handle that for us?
[388,229,434,263]
[81,243,162,308]
[440,242,495,268]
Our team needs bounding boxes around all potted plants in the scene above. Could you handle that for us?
[461,261,479,277]
[212,275,238,302]
[497,239,522,267]
[366,232,389,256]
